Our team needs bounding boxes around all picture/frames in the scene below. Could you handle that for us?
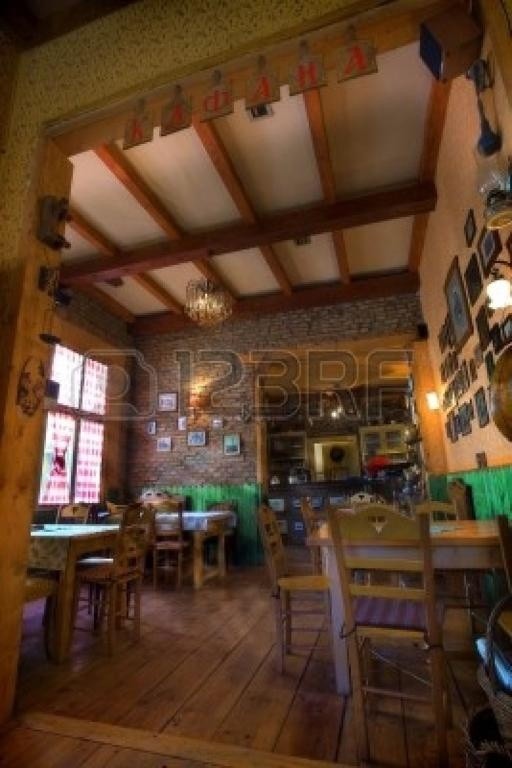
[222,432,242,456]
[157,392,177,412]
[436,206,512,443]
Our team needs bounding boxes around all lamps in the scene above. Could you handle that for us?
[185,258,237,324]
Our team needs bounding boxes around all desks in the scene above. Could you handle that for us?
[304,520,508,697]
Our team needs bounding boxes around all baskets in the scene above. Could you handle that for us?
[475,594,512,741]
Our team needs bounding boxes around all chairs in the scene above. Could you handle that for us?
[255,477,474,766]
[24,489,238,661]
